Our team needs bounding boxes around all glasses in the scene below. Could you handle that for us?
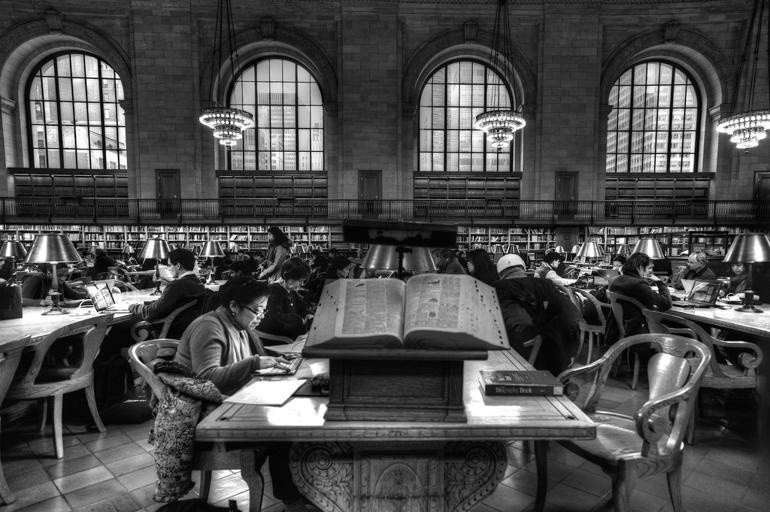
[245,304,268,316]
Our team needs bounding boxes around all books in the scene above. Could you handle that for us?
[0,224,331,255]
[252,334,307,376]
[649,279,718,307]
[302,275,510,357]
[478,369,564,396]
[457,225,764,262]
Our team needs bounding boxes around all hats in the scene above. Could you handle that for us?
[497,254,526,276]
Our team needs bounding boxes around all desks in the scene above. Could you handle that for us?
[0,279,228,395]
[652,284,770,442]
[193,330,597,511]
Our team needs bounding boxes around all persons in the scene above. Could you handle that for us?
[21,247,115,307]
[130,228,351,356]
[431,249,748,379]
[171,274,306,512]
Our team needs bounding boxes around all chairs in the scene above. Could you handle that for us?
[609,290,649,392]
[129,337,267,512]
[569,282,610,366]
[8,313,118,460]
[255,328,292,350]
[642,306,766,447]
[127,298,199,407]
[530,333,711,512]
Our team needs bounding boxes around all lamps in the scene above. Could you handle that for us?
[473,0,527,155]
[26,232,83,315]
[1,240,28,270]
[357,246,437,278]
[715,18,770,154]
[722,232,769,314]
[196,1,257,151]
[136,237,171,296]
